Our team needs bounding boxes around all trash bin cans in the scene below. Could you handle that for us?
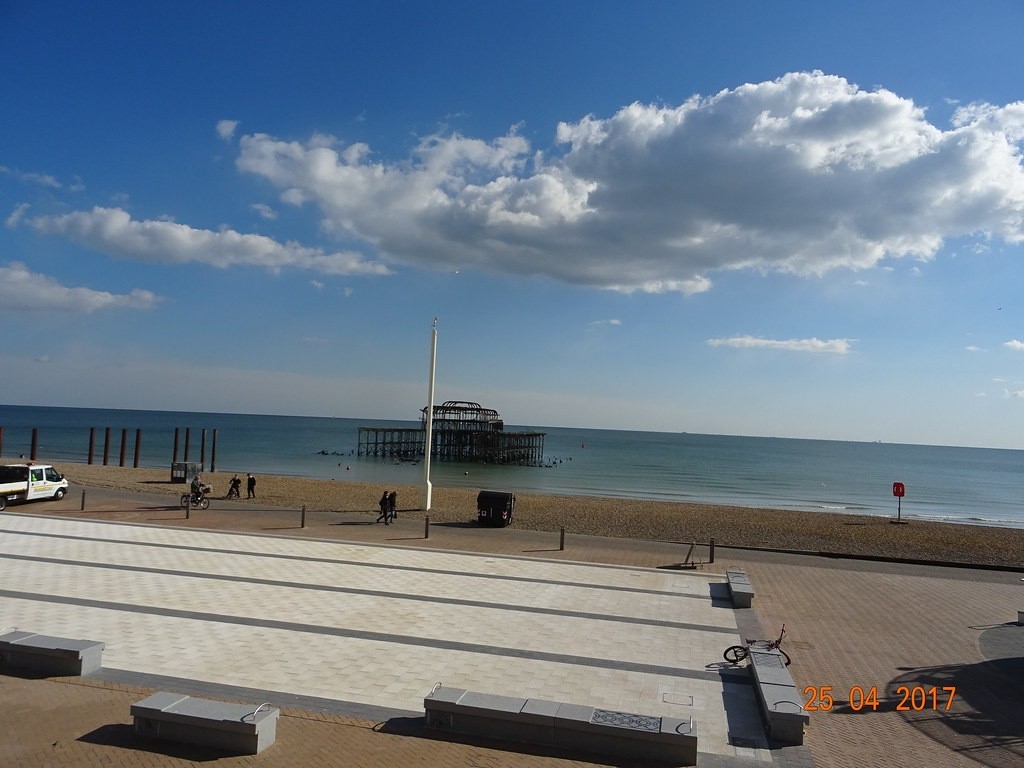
[171,462,202,483]
[477,490,515,528]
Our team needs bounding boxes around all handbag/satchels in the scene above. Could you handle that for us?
[394,510,397,519]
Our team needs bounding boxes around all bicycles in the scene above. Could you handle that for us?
[723,623,790,668]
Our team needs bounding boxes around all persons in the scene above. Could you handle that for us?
[247,472,256,499]
[377,491,397,525]
[226,474,242,498]
[191,475,203,502]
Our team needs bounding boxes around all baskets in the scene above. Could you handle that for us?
[203,488,214,493]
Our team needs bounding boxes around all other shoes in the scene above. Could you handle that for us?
[377,519,379,523]
[385,522,389,525]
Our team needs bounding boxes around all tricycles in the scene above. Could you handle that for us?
[180,483,210,510]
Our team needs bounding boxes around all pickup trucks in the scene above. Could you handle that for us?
[0,464,69,511]
